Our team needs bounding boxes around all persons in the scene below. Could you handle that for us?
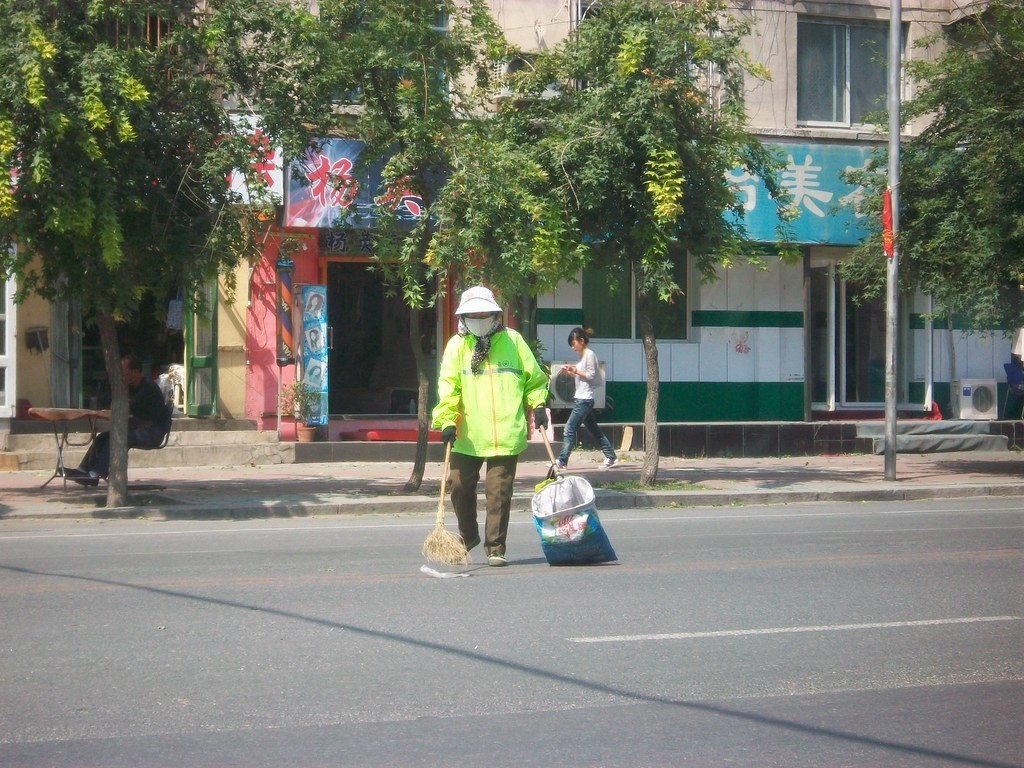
[308,398,321,414]
[551,328,621,471]
[58,352,167,486]
[309,329,320,352]
[306,294,323,315]
[431,287,548,565]
[308,365,320,383]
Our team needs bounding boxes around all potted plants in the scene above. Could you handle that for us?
[277,380,321,441]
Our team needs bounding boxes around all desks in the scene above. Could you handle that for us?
[29,407,112,489]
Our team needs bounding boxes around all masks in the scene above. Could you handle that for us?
[464,313,496,337]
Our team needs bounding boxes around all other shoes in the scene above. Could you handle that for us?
[488,553,507,566]
[58,467,99,487]
[599,458,619,470]
[459,536,481,552]
[546,459,567,470]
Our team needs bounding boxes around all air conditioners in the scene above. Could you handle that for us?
[493,50,562,103]
[950,378,998,420]
[542,360,606,407]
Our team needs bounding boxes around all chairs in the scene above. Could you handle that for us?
[126,403,174,491]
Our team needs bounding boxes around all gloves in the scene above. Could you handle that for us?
[442,425,456,447]
[534,407,548,432]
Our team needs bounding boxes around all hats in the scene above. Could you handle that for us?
[454,286,503,335]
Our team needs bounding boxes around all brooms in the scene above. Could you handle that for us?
[421,440,475,570]
